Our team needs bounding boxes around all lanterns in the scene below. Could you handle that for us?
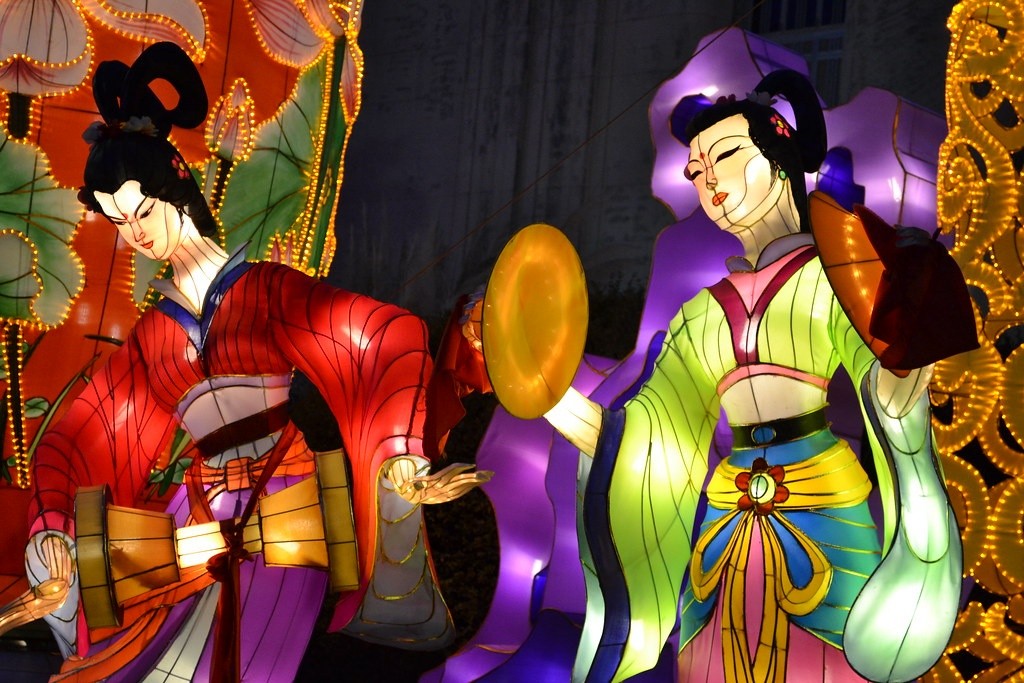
[0,0,1024,683]
